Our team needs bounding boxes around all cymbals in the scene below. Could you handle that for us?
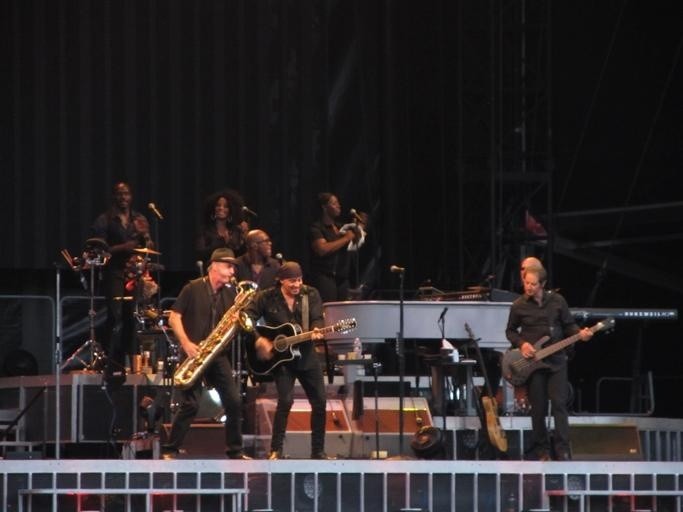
[133,247,159,255]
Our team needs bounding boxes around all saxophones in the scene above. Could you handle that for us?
[173,276,260,392]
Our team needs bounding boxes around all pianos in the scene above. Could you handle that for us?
[419,288,523,300]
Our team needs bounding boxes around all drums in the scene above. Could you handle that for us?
[139,323,179,373]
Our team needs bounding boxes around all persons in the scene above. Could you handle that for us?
[198,189,281,290]
[92,181,154,367]
[245,262,337,459]
[520,257,542,279]
[309,193,368,302]
[505,264,593,461]
[162,248,252,459]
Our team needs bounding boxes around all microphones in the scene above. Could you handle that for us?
[389,265,410,275]
[148,202,164,221]
[241,206,259,219]
[438,306,448,322]
[195,260,204,279]
[350,208,364,223]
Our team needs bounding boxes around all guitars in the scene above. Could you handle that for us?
[244,318,357,379]
[464,321,508,453]
[503,318,615,386]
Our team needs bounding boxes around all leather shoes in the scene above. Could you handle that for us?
[311,453,332,459]
[267,451,280,459]
[162,453,173,460]
[230,453,253,459]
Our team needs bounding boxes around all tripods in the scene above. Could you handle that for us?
[60,279,106,372]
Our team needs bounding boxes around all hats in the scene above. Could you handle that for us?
[272,262,302,280]
[210,248,240,264]
